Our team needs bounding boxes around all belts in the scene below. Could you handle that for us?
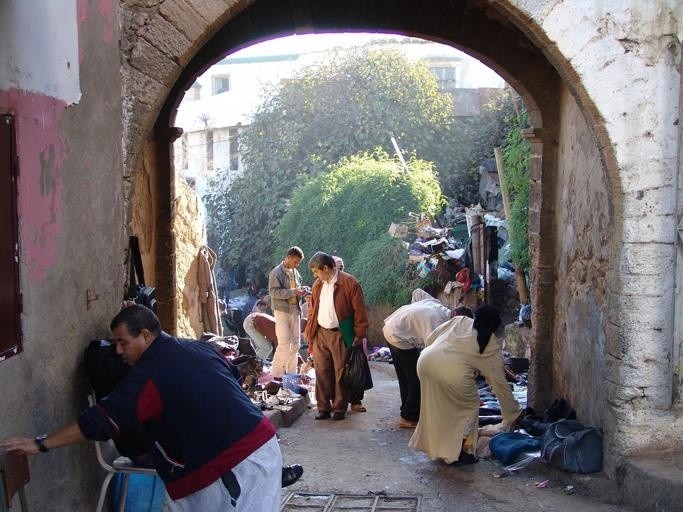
[330,327,338,331]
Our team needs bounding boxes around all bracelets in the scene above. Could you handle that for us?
[34,432,49,452]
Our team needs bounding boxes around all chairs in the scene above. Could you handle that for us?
[0,450,32,512]
[78,379,159,512]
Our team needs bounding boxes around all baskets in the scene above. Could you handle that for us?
[282,373,301,393]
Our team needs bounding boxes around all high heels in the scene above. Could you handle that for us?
[455,453,479,463]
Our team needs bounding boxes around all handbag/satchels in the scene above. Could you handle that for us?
[135,287,158,314]
[510,398,602,473]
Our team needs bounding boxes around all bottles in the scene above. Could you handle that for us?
[291,374,301,393]
[281,374,291,389]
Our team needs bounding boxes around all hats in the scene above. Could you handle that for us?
[331,256,344,271]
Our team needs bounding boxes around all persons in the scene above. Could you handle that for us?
[407,306,519,465]
[217,283,313,376]
[78,299,303,488]
[302,253,369,420]
[0,302,282,512]
[331,254,368,411]
[382,287,474,428]
[269,246,312,383]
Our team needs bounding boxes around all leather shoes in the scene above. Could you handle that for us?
[282,464,303,488]
[315,404,366,420]
